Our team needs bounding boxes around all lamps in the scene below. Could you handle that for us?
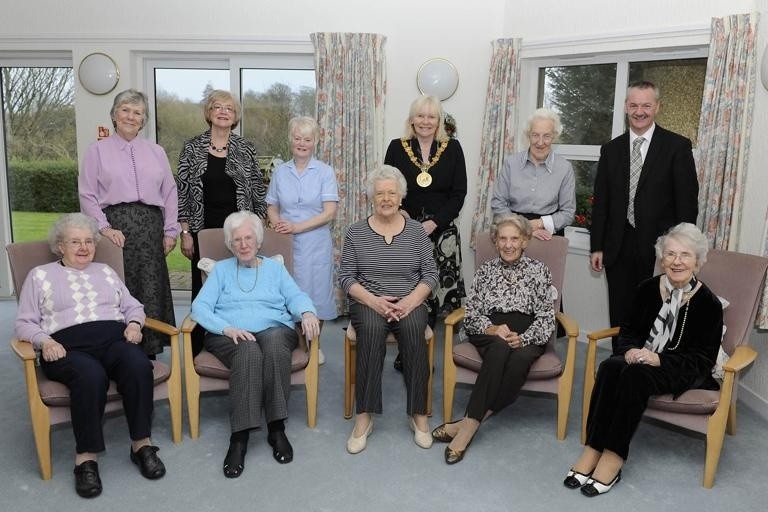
[416,56,460,103]
[76,52,123,98]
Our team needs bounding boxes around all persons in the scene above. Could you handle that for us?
[432,212,557,464]
[78,88,182,361]
[13,212,166,497]
[190,211,321,477]
[490,109,577,338]
[384,94,467,373]
[338,164,442,454]
[175,90,268,360]
[563,222,723,497]
[265,117,339,365]
[588,81,699,356]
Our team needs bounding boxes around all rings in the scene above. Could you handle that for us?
[628,361,631,364]
[639,358,644,364]
[510,342,514,345]
[113,234,115,236]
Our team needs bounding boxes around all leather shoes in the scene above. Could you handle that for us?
[409,416,433,449]
[347,415,373,454]
[564,468,621,497]
[74,460,102,497]
[433,418,463,442]
[224,439,246,477]
[130,445,165,478]
[268,432,293,464]
[445,423,480,464]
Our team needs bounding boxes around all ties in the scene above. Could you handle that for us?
[626,136,646,229]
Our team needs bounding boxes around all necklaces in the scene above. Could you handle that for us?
[400,136,448,187]
[237,259,259,292]
[210,142,228,153]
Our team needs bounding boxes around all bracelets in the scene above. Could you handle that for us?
[181,231,191,234]
[129,320,141,328]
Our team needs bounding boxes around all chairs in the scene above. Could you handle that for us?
[4,224,186,480]
[183,222,323,443]
[438,225,581,444]
[580,244,767,491]
[342,311,439,427]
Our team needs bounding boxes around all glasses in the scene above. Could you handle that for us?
[211,104,235,114]
[664,252,698,263]
[60,238,98,248]
